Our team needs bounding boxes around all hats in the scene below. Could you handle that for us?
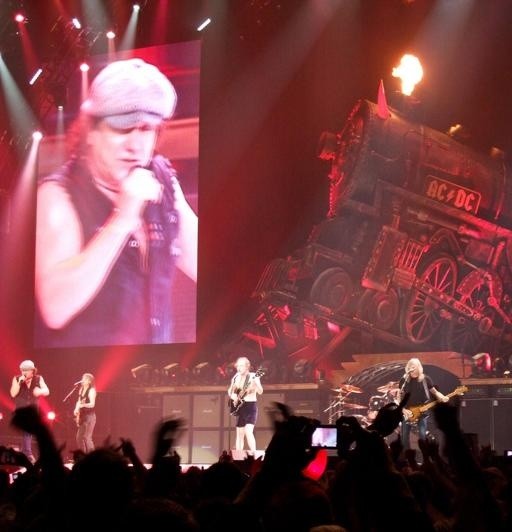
[20,360,34,371]
[80,58,178,130]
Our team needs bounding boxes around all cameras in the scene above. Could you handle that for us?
[310,425,342,449]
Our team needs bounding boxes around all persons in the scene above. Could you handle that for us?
[1,389,512,532]
[32,57,201,350]
[227,354,265,452]
[397,356,452,453]
[9,359,52,465]
[72,371,98,454]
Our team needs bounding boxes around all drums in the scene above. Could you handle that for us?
[367,396,388,419]
[349,414,367,428]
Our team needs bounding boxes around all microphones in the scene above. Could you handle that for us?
[19,375,26,383]
[406,369,414,375]
[234,374,240,380]
[74,381,84,385]
[129,165,164,245]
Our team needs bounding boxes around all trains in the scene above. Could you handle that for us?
[252,78,512,354]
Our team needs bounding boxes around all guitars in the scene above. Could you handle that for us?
[228,364,269,416]
[76,397,86,427]
[403,386,468,427]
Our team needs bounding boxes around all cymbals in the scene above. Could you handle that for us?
[330,388,352,396]
[377,381,400,392]
[341,384,364,394]
[342,403,367,409]
